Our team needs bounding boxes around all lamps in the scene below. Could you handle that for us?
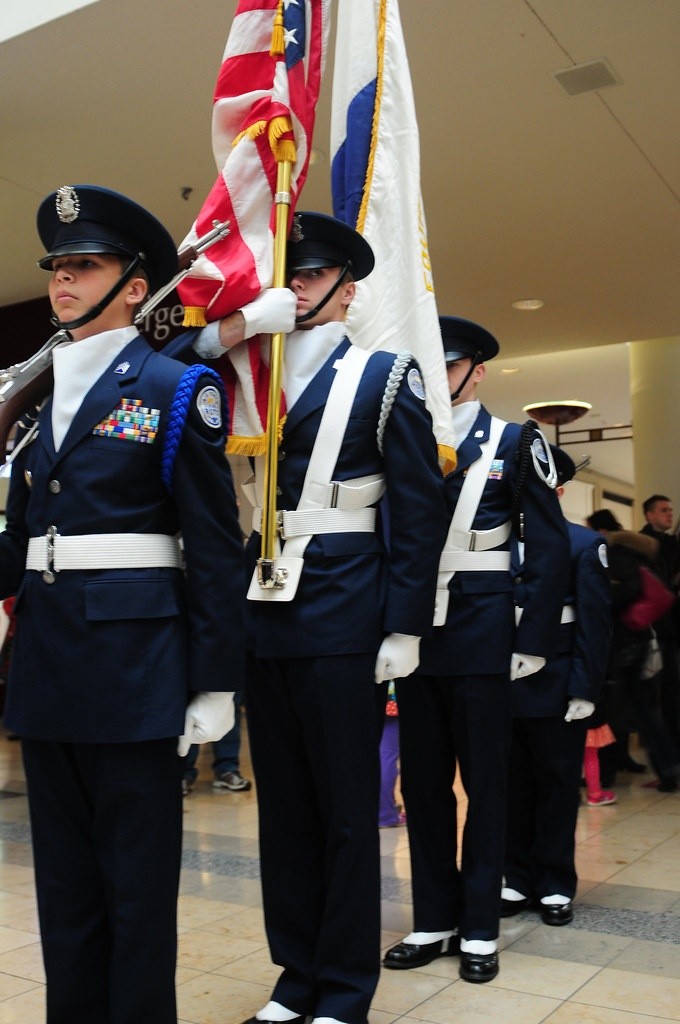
[522,400,632,447]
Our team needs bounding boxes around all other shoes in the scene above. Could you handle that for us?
[602,768,616,786]
[620,758,646,772]
[378,813,407,827]
[657,784,675,791]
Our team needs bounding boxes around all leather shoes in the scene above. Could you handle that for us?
[458,941,499,980]
[539,894,574,924]
[242,1013,313,1024]
[502,885,532,915]
[382,934,460,966]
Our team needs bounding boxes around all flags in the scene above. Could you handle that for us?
[331,1,457,474]
[177,0,333,457]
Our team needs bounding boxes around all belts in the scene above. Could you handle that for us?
[438,551,512,571]
[251,506,376,541]
[513,604,577,627]
[25,532,185,571]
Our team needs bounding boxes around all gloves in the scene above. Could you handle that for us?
[510,653,546,679]
[374,632,422,684]
[177,691,235,757]
[564,697,595,722]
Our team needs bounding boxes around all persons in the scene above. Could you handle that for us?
[165,210,446,1023]
[499,444,611,926]
[183,706,252,794]
[386,315,574,982]
[586,493,680,805]
[0,184,245,1024]
[379,680,407,827]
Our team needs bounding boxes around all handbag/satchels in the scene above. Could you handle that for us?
[639,624,663,680]
[621,561,677,631]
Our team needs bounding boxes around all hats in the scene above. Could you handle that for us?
[539,443,576,486]
[288,212,375,280]
[438,316,499,362]
[35,185,180,284]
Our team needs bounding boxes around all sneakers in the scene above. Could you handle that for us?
[587,790,618,805]
[212,771,251,789]
[181,779,196,794]
